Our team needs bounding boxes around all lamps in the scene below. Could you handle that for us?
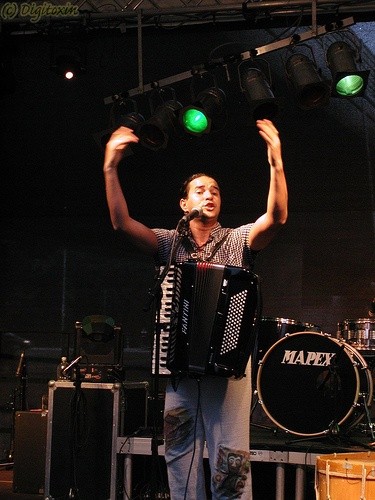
[109,30,371,152]
[75,315,124,365]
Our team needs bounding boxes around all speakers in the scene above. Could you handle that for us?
[11,413,48,495]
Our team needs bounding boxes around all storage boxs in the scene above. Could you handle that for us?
[45,379,149,500]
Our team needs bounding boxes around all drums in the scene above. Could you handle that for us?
[252,315,320,395]
[341,317,375,357]
[257,331,373,437]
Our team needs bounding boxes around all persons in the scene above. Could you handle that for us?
[104,119,288,500]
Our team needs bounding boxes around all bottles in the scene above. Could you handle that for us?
[57,357,69,380]
[42,393,48,410]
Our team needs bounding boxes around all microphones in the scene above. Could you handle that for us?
[15,351,24,376]
[180,207,203,223]
